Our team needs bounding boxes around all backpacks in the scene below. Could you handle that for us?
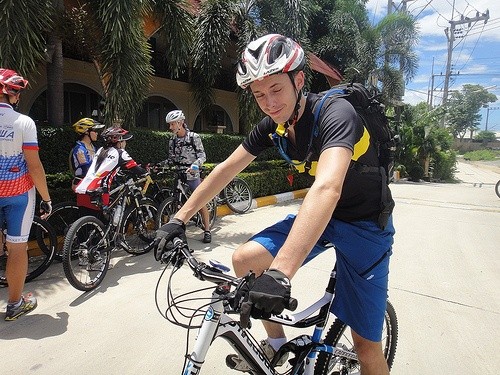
[276,82,401,173]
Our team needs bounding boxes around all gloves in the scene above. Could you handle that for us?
[240,268,292,328]
[39,200,52,220]
[154,218,188,269]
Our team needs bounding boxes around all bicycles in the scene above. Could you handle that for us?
[0,203,57,288]
[39,166,160,291]
[160,237,399,375]
[194,169,252,230]
[148,165,217,232]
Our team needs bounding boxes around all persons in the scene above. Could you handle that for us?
[152,109,211,243]
[76,126,150,272]
[69,118,107,265]
[152,34,395,375]
[0,68,52,319]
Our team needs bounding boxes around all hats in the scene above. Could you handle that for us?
[92,124,105,129]
[119,134,134,141]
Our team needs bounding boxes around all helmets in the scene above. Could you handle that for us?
[101,126,129,142]
[0,68,28,96]
[72,117,100,135]
[236,33,306,89]
[166,109,185,123]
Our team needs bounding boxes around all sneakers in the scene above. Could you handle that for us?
[203,231,212,243]
[78,256,89,265]
[85,260,114,271]
[226,340,290,372]
[4,295,38,321]
